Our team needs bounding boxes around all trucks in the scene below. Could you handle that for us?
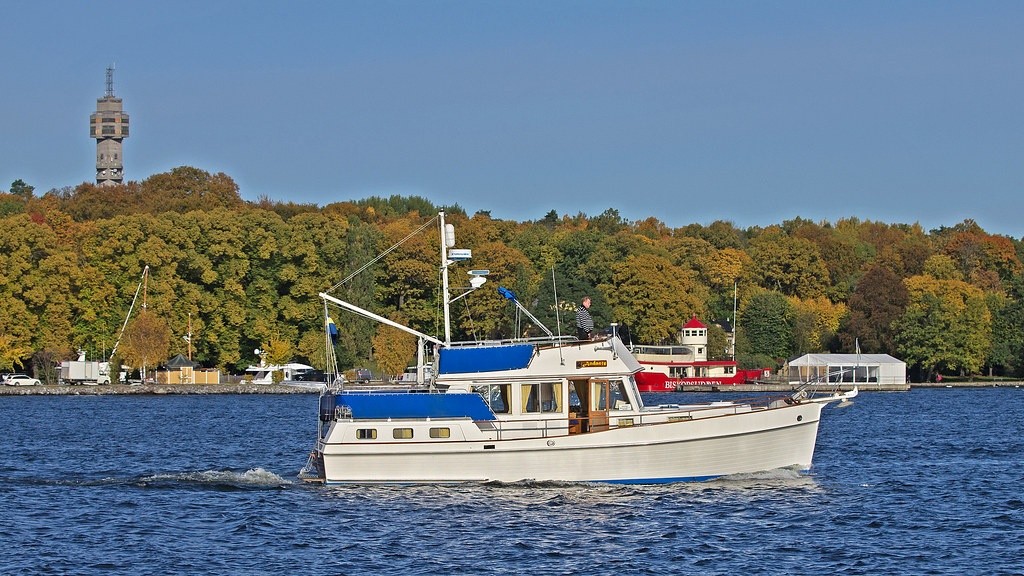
[60,360,112,386]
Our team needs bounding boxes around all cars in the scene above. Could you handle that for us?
[4,374,42,387]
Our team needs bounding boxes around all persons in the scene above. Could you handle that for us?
[575,295,594,340]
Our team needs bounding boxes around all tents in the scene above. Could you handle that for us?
[789,354,879,385]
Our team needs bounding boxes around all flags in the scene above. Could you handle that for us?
[325,314,337,346]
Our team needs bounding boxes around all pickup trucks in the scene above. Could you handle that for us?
[296,369,334,383]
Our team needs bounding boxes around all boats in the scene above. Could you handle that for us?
[397,344,440,388]
[624,280,762,393]
[299,210,861,486]
[238,345,315,385]
